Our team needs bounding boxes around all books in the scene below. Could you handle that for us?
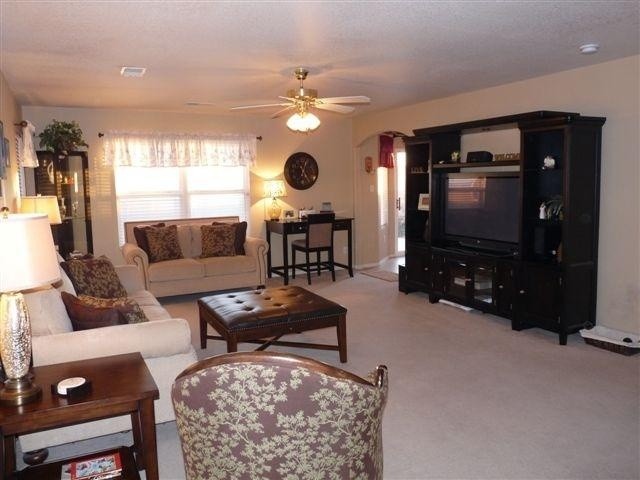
[453,275,492,291]
[59,453,123,479]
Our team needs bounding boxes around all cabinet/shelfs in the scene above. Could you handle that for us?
[399,110,608,346]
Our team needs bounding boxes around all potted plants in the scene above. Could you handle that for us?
[40,119,87,217]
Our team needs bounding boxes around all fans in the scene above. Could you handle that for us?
[228,68,371,116]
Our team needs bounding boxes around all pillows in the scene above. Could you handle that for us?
[59,256,149,331]
[134,220,247,262]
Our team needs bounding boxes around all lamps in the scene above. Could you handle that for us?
[263,180,288,220]
[19,194,62,226]
[0,207,65,408]
[285,112,320,131]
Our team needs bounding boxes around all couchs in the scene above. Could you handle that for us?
[122,216,270,298]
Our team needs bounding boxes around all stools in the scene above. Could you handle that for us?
[198,285,347,364]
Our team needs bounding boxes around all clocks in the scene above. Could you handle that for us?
[284,152,319,190]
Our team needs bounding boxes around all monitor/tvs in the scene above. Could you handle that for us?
[440,171,523,253]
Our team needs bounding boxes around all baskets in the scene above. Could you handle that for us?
[581,321,640,355]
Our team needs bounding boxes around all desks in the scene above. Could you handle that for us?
[263,214,354,285]
[0,352,160,480]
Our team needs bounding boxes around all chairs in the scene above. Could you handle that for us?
[291,213,335,285]
[171,351,389,480]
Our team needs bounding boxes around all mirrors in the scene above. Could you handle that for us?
[34,150,92,256]
